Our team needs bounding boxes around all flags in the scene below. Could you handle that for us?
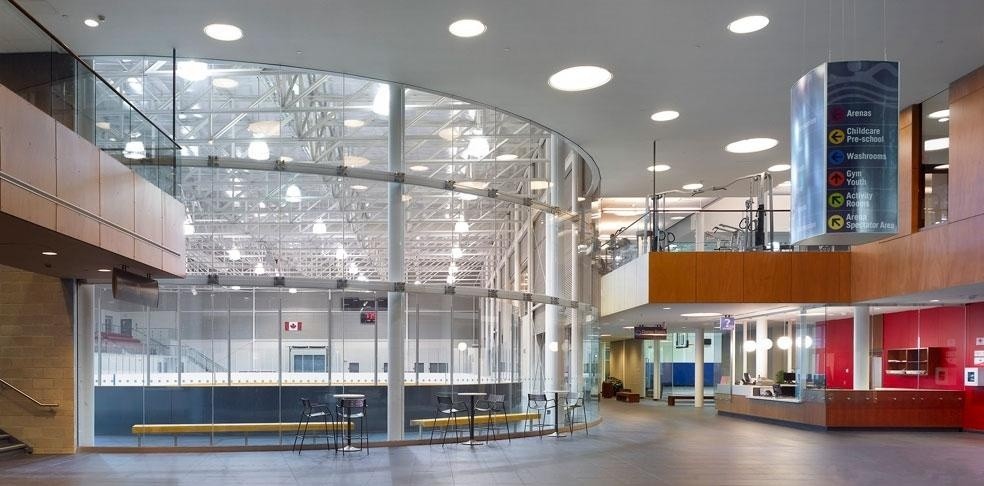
[284,321,302,332]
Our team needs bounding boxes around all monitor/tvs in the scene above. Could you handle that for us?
[805,373,825,387]
[783,372,795,384]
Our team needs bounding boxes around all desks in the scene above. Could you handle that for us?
[333,394,365,452]
[523,393,559,439]
[544,390,571,437]
[458,393,488,445]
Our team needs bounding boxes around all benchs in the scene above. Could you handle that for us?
[410,413,541,440]
[132,422,355,447]
[616,392,640,402]
[668,396,716,406]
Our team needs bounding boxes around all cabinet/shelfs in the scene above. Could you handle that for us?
[885,347,934,376]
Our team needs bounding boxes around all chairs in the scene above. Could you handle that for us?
[335,398,369,455]
[292,397,338,455]
[474,394,512,441]
[430,396,470,447]
[562,393,589,436]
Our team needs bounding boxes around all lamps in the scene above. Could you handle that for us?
[743,311,812,352]
[97,58,519,283]
